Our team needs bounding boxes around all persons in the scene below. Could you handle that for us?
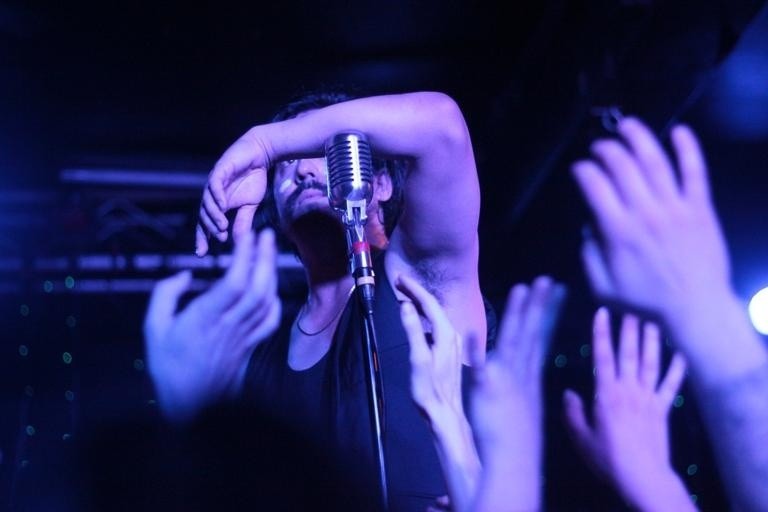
[465,276,569,512]
[560,308,700,512]
[569,114,768,512]
[192,91,500,512]
[139,225,286,437]
[385,271,492,512]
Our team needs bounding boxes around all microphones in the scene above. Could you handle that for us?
[325,125,375,318]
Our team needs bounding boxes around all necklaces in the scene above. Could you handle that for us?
[296,284,357,338]
